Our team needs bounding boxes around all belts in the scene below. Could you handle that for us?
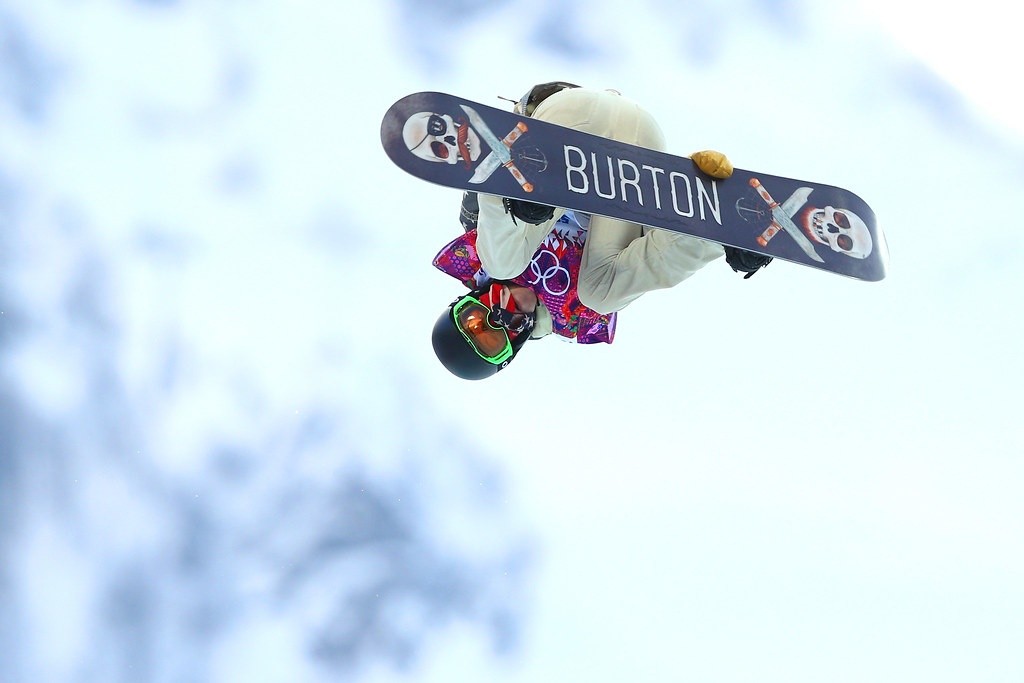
[522,82,582,117]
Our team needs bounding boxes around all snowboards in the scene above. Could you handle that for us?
[380,91,892,282]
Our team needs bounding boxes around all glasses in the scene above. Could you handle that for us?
[453,295,513,364]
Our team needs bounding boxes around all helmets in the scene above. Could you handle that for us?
[432,279,536,382]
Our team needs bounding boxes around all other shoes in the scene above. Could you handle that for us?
[722,245,774,280]
[501,196,556,224]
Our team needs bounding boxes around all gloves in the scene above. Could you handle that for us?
[689,150,733,179]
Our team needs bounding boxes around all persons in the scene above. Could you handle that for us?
[428,79,776,381]
